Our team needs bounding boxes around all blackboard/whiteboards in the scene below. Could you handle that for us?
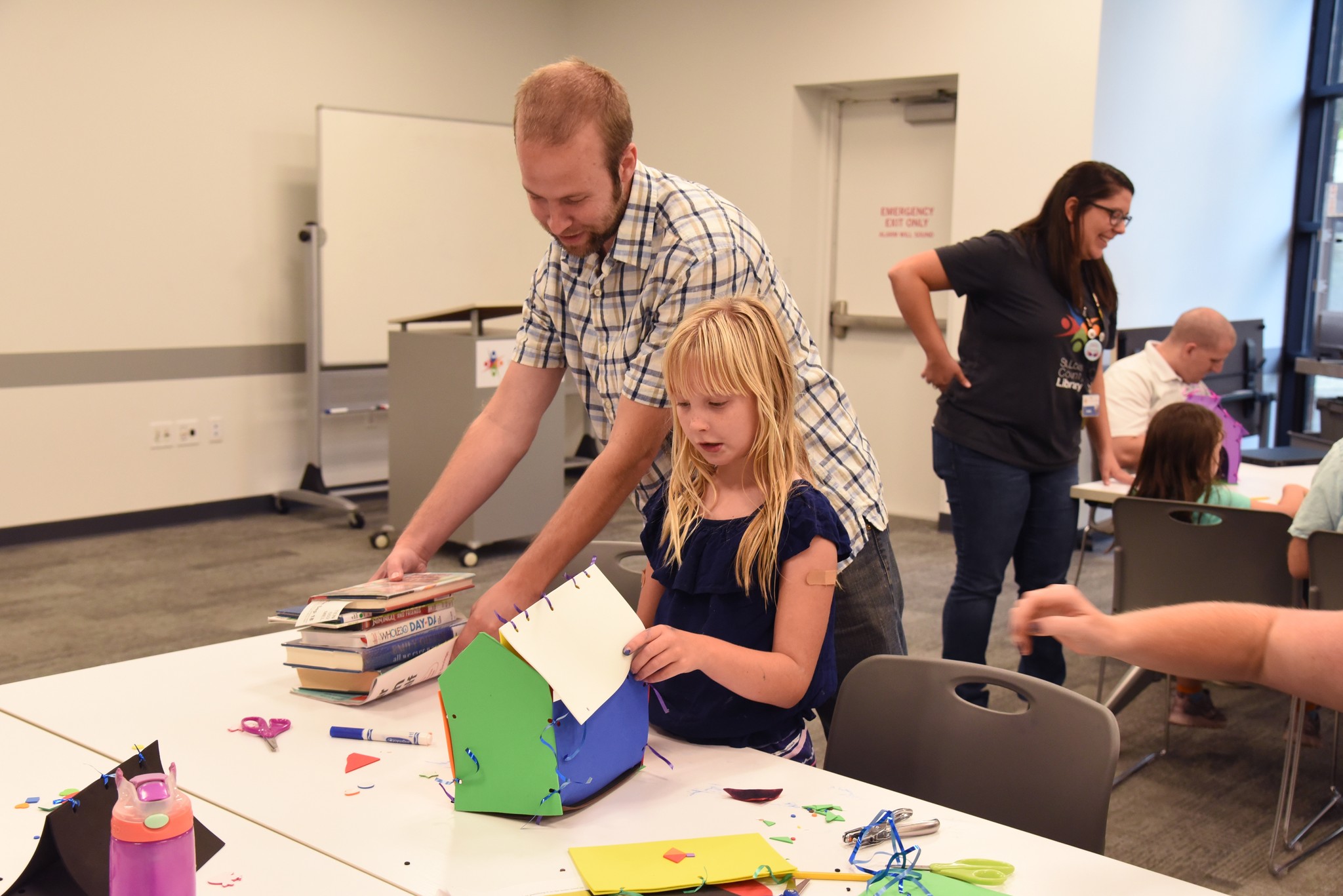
[299,103,555,420]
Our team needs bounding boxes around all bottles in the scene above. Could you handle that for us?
[109,762,196,896]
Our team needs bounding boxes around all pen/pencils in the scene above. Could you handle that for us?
[770,871,874,882]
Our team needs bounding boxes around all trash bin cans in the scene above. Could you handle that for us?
[371,305,567,568]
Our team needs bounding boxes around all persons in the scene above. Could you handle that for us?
[889,160,1134,709]
[1008,584,1343,713]
[364,57,910,741]
[1102,400,1308,728]
[1088,306,1236,471]
[1285,437,1343,579]
[621,294,853,771]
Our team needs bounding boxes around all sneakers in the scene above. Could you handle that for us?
[1168,688,1229,729]
[1283,709,1326,748]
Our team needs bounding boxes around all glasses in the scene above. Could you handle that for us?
[1086,201,1132,227]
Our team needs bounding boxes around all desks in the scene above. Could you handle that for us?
[0,712,413,896]
[0,628,1229,896]
[1070,462,1318,710]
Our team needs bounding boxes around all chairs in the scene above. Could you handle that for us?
[826,418,1343,856]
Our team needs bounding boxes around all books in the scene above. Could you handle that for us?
[266,570,478,694]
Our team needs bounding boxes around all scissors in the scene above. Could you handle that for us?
[241,716,291,751]
[885,858,1015,885]
[778,876,811,896]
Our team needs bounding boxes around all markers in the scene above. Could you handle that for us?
[325,407,349,415]
[329,726,433,746]
